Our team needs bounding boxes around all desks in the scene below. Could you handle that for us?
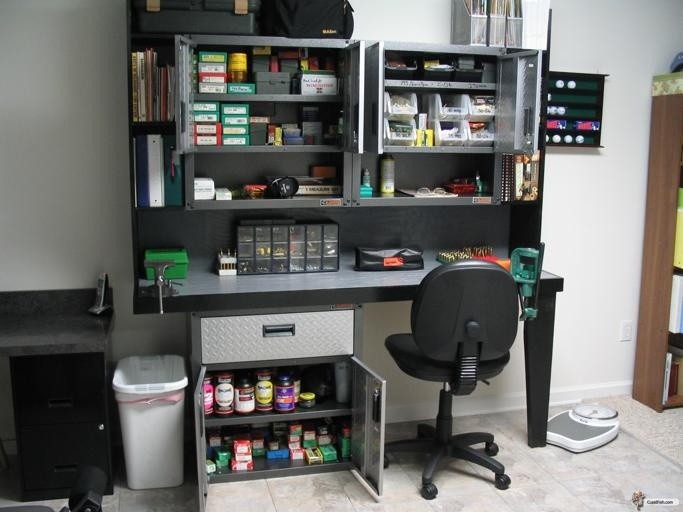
[1,285,119,503]
[132,249,563,450]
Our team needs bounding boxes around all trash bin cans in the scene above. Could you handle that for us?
[112,354,188,491]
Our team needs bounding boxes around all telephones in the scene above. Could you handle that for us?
[88,273,112,314]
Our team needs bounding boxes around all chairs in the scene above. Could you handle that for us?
[383,259,520,501]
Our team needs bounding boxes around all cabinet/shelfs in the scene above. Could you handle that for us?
[186,302,387,512]
[631,72,682,413]
[131,34,366,215]
[544,70,610,150]
[353,34,543,209]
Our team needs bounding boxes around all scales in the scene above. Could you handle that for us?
[546,404,620,454]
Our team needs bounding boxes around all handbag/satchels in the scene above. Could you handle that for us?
[259,0,354,38]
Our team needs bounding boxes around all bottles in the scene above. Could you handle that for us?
[340,428,351,458]
[380,152,395,194]
[363,168,371,187]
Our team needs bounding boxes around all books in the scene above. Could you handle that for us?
[129,46,178,123]
[667,359,678,398]
[673,352,683,398]
[659,352,673,405]
